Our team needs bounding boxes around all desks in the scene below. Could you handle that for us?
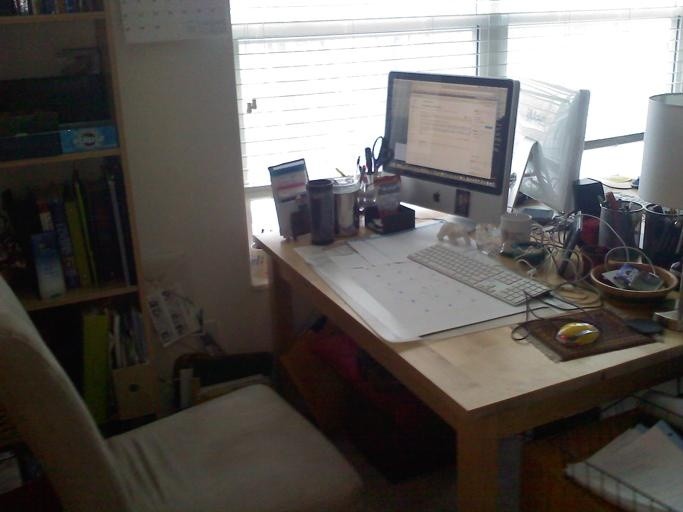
[249,230,681,512]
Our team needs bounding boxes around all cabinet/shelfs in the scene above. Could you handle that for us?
[0,0,157,430]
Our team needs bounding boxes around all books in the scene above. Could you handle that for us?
[64,307,160,423]
[0,0,101,15]
[563,419,682,509]
[1,171,137,301]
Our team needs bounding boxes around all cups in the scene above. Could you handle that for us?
[498,213,531,254]
[305,176,360,245]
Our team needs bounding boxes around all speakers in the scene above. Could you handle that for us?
[573,178,605,218]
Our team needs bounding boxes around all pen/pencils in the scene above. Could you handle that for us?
[606,191,683,271]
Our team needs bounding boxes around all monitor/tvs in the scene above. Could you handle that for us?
[506,76,590,231]
[382,71,520,226]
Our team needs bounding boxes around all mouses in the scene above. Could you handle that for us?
[556,322,601,345]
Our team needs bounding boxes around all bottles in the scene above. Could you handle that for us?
[372,175,401,225]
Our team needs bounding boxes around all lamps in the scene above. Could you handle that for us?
[636,93,682,334]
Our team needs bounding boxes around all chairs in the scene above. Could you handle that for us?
[0,280,369,512]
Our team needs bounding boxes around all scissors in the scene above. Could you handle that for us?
[372,136,394,179]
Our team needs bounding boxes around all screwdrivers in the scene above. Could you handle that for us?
[365,147,372,185]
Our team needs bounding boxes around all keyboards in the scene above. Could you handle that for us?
[408,244,552,306]
